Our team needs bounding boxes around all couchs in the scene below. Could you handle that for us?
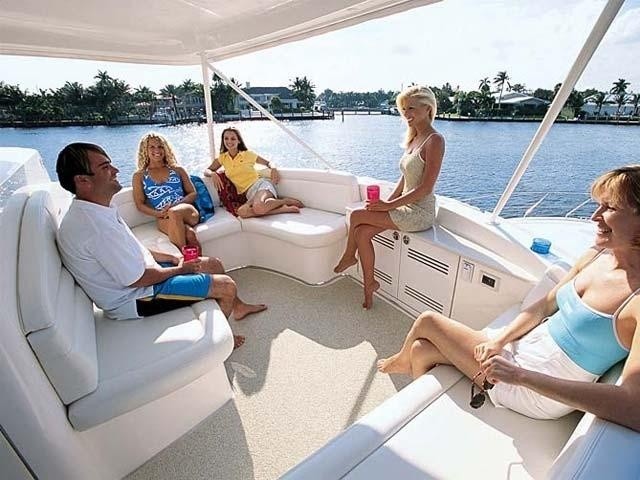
[268,273,640,480]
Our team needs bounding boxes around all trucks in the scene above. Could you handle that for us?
[0,108,16,123]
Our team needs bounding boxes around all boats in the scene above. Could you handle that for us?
[0,0,640,480]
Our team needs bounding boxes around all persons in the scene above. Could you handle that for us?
[132,133,203,260]
[202,128,305,219]
[333,86,448,311]
[377,164,639,437]
[53,141,268,349]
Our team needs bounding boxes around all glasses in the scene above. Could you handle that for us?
[470,372,495,409]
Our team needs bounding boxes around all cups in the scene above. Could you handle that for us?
[531,237,552,254]
[182,245,197,263]
[367,185,380,201]
